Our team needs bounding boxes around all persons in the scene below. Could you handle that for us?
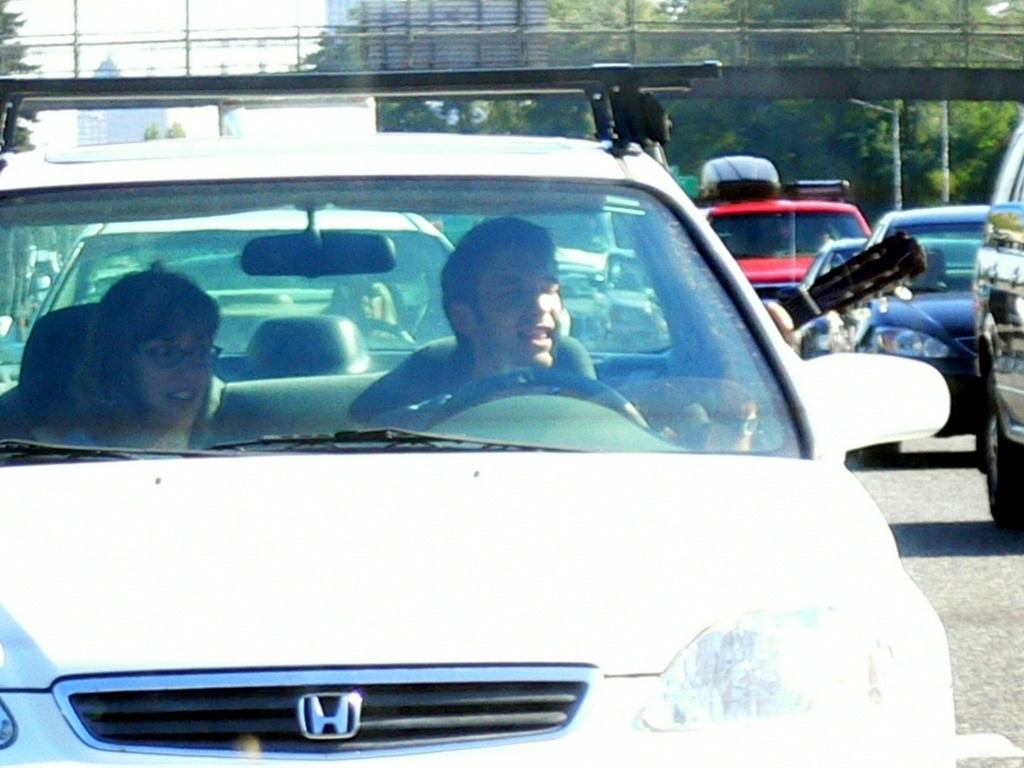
[23,258,224,459]
[817,230,835,251]
[328,272,417,345]
[372,218,799,452]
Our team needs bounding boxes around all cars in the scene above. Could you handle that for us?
[549,151,993,464]
[0,206,460,388]
[1,62,963,764]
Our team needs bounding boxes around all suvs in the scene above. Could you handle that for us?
[969,111,1024,538]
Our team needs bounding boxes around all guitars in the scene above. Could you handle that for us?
[784,229,928,330]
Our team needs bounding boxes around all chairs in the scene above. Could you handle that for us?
[365,336,597,423]
[0,301,222,458]
[224,315,384,449]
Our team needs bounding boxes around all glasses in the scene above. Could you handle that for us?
[136,343,225,368]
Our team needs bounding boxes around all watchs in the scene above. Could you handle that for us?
[716,412,759,436]
